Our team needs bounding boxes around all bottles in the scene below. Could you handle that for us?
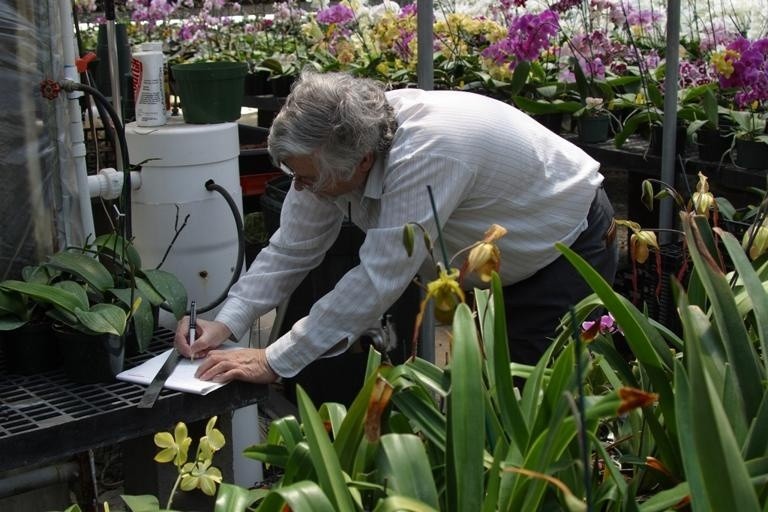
[129,41,167,127]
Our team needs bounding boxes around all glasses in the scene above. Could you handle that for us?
[278,161,320,189]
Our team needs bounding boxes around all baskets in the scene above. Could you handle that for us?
[639,238,692,338]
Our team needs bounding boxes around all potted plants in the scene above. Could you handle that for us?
[0,232,191,386]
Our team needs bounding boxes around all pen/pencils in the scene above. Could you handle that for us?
[190,300,196,361]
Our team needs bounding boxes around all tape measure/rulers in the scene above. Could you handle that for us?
[137,347,182,409]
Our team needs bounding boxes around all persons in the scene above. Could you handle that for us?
[172,72,622,398]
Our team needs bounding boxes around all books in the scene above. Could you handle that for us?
[115,344,253,397]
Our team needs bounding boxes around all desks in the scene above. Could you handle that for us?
[1,325,275,512]
[565,133,768,271]
